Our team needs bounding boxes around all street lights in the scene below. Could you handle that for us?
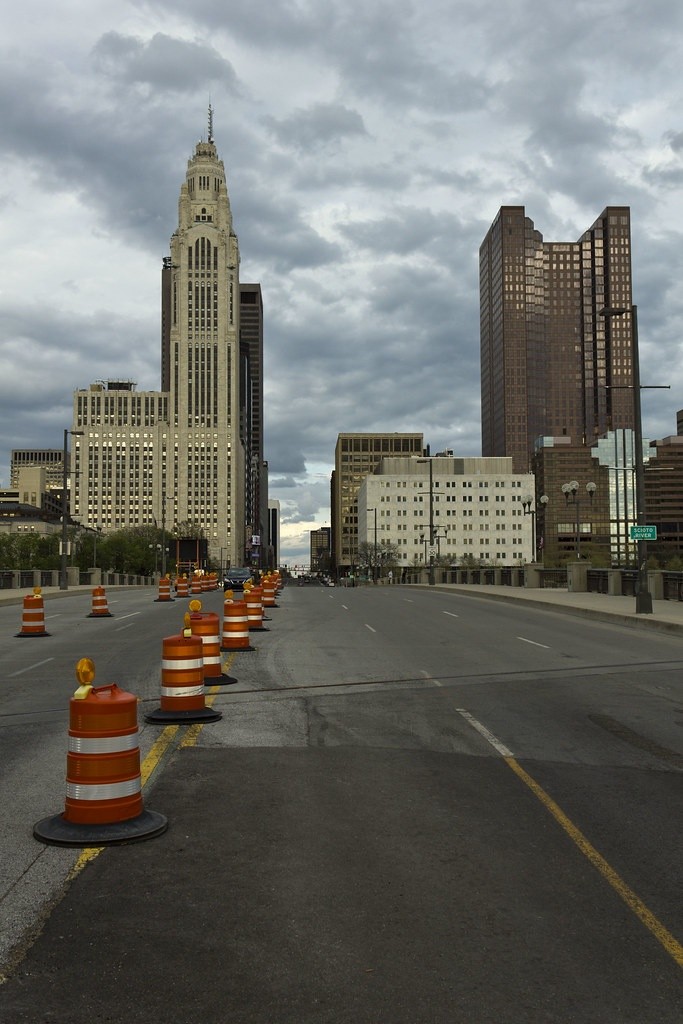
[60,429,85,590]
[366,508,377,584]
[149,543,162,575]
[417,458,436,585]
[160,491,175,579]
[521,495,550,561]
[200,528,210,578]
[221,547,227,588]
[598,304,654,614]
[562,481,598,560]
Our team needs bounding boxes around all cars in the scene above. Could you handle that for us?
[223,566,254,593]
